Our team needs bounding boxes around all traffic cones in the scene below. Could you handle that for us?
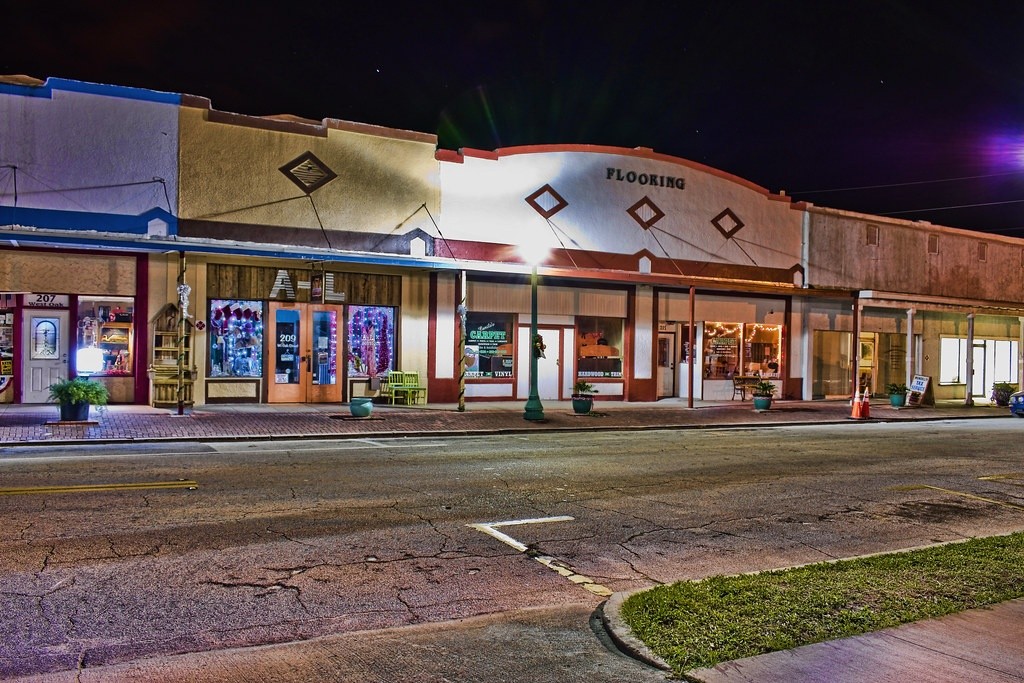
[862,386,871,418]
[851,388,862,418]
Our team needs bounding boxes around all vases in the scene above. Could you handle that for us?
[350,398,373,416]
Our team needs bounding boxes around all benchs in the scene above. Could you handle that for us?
[732,376,762,401]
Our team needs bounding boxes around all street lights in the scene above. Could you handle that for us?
[522,236,544,421]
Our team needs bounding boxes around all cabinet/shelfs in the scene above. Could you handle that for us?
[148,303,197,409]
[0,327,13,347]
[96,321,133,344]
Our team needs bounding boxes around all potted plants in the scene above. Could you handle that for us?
[41,377,111,422]
[752,382,776,409]
[990,381,1017,406]
[570,381,598,413]
[886,383,911,407]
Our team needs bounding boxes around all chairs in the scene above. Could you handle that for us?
[387,371,427,406]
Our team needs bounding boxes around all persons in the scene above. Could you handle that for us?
[859,372,867,386]
